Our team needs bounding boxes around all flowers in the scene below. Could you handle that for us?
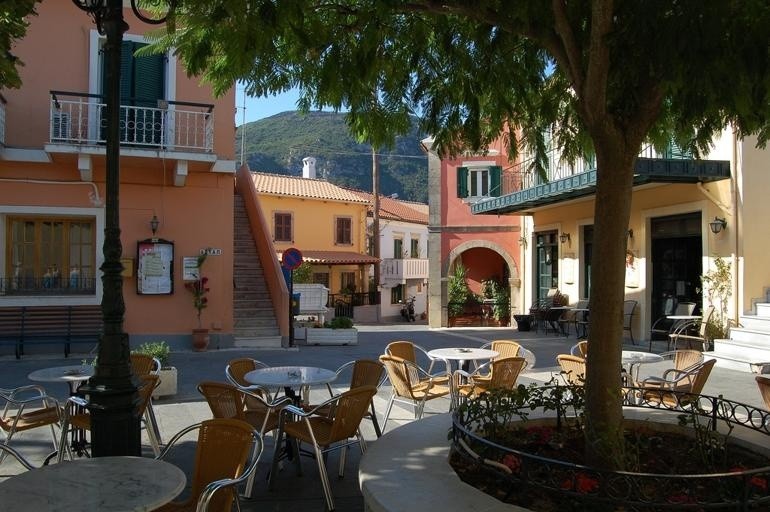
[184,252,210,328]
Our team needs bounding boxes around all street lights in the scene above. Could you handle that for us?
[372,192,399,300]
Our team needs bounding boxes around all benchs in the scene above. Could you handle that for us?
[0,305,104,360]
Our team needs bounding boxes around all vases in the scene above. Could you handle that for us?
[192,328,210,352]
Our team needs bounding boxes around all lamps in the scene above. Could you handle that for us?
[149,216,160,236]
[560,232,570,243]
[518,236,526,246]
[709,216,727,235]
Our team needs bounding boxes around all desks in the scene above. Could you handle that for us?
[665,314,703,359]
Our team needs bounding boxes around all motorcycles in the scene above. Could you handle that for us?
[398,295,417,322]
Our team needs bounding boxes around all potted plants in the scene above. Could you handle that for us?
[306,316,359,346]
[696,253,731,351]
[493,294,510,327]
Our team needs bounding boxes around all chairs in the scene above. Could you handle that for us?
[529,288,589,339]
[649,302,697,355]
[669,306,714,363]
[623,300,639,346]
